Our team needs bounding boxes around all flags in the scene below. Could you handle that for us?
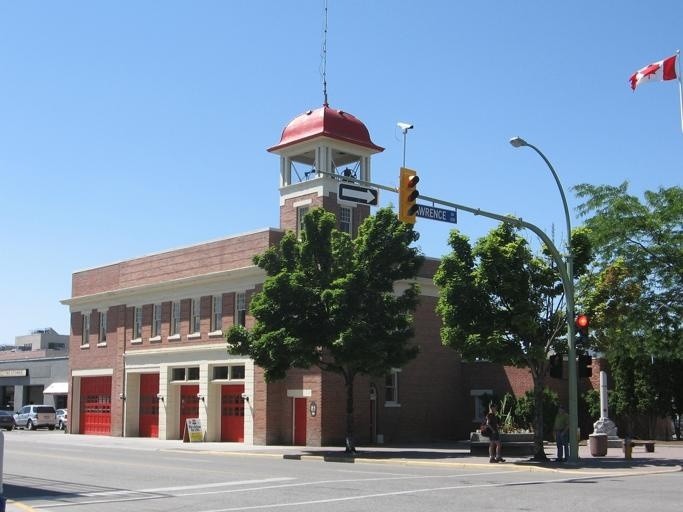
[628,55,678,94]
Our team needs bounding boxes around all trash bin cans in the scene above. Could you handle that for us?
[589,433,607,456]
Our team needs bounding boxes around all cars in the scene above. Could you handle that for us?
[0,404,68,430]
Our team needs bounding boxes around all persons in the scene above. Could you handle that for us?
[486,403,506,464]
[553,406,570,462]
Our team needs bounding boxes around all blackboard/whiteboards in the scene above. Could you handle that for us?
[187,418,204,441]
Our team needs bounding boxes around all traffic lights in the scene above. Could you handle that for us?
[399,167,419,224]
[575,311,588,346]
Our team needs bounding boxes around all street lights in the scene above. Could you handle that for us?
[509,136,582,462]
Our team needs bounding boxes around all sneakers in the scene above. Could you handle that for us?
[557,458,568,463]
[490,457,506,463]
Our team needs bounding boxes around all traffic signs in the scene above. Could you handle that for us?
[416,203,457,224]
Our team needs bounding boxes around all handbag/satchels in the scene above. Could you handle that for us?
[480,425,490,437]
